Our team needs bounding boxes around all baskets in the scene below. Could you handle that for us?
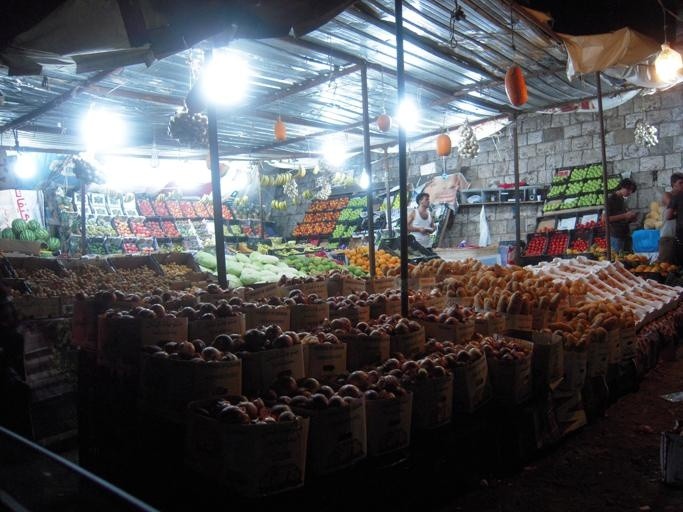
[291,188,447,248]
[221,217,283,249]
[377,235,440,266]
[518,163,624,264]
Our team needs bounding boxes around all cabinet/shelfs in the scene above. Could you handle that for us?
[456,183,547,207]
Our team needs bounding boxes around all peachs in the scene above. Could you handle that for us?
[616,254,678,275]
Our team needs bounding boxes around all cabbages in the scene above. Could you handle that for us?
[193,251,298,288]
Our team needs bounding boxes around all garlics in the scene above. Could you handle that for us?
[634,121,659,148]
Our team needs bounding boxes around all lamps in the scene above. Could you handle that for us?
[654,8,682,79]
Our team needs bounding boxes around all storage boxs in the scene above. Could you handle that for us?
[0,237,637,511]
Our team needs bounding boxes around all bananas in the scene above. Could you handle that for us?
[271,191,313,209]
[260,164,306,186]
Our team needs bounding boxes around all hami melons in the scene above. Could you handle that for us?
[644,202,664,229]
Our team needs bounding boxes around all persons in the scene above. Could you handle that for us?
[406,193,437,253]
[602,179,638,255]
[661,172,681,240]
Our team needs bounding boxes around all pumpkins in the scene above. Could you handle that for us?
[504,64,529,106]
[377,115,390,132]
[274,122,285,142]
[436,134,451,156]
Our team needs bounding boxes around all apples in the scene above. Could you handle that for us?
[76,283,531,426]
[332,198,366,237]
[543,163,621,212]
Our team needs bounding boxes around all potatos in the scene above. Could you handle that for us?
[382,257,635,352]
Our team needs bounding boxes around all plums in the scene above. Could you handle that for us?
[113,199,233,253]
[524,220,608,257]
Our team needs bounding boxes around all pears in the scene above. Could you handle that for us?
[287,256,366,278]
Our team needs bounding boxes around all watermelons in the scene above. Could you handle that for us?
[0,219,60,251]
